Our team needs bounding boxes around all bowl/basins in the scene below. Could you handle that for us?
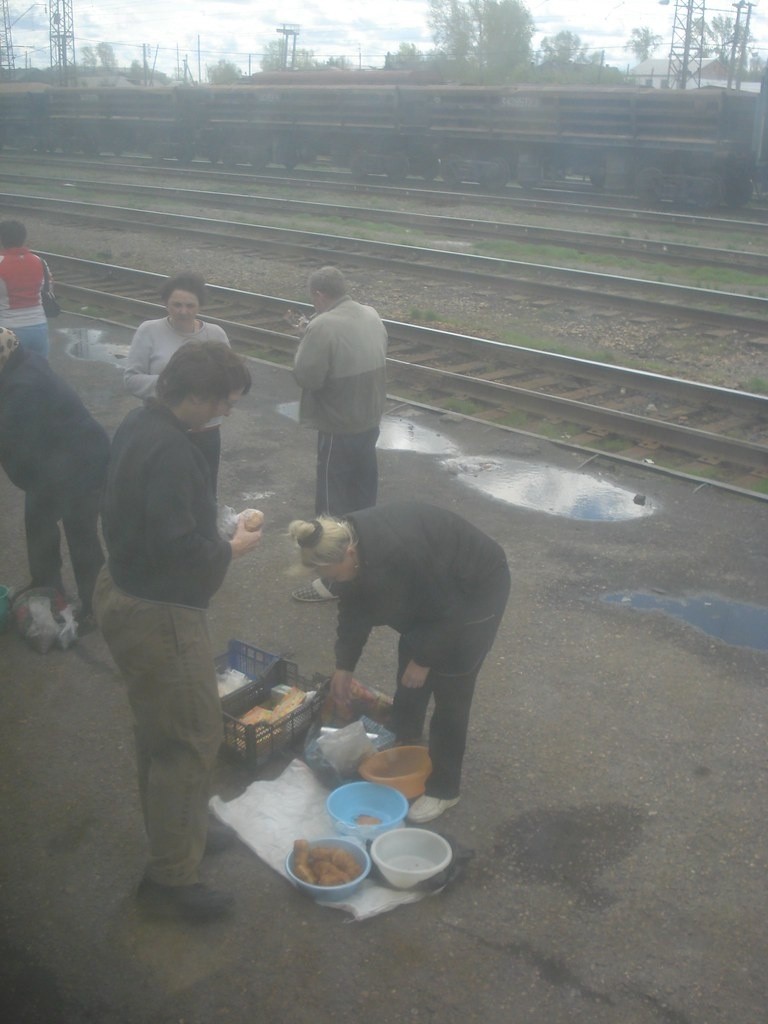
[285,838,372,900]
[358,745,432,799]
[326,781,409,839]
[370,827,454,889]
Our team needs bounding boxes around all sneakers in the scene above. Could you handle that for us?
[406,795,460,823]
[136,879,234,920]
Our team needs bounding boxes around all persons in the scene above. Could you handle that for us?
[91,269,263,920]
[293,265,388,602]
[288,500,512,824]
[0,219,111,638]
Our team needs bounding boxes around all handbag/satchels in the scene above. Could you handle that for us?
[39,258,61,318]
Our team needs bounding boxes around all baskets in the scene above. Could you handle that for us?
[207,639,332,771]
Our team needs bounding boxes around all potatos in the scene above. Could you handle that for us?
[292,838,361,887]
[234,508,264,531]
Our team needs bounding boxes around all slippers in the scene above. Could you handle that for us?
[292,578,340,602]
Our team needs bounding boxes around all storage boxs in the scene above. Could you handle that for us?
[213,637,332,775]
